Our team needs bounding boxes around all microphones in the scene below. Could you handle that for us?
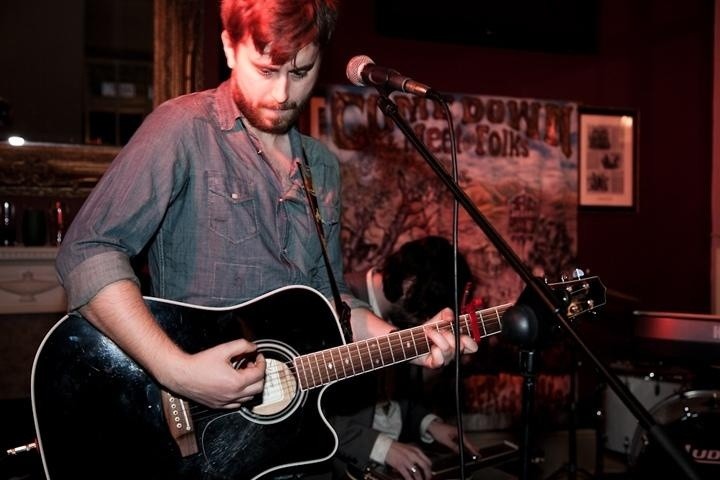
[343,50,429,102]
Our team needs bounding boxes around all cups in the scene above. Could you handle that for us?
[22,208,47,246]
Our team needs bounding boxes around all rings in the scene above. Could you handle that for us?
[410,465,419,474]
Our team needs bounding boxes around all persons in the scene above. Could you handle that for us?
[322,234,482,480]
[53,0,482,479]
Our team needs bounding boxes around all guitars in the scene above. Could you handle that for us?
[31,268,608,480]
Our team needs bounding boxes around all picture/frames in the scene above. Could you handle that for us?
[574,102,643,215]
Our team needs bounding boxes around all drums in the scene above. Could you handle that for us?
[632,390,720,480]
